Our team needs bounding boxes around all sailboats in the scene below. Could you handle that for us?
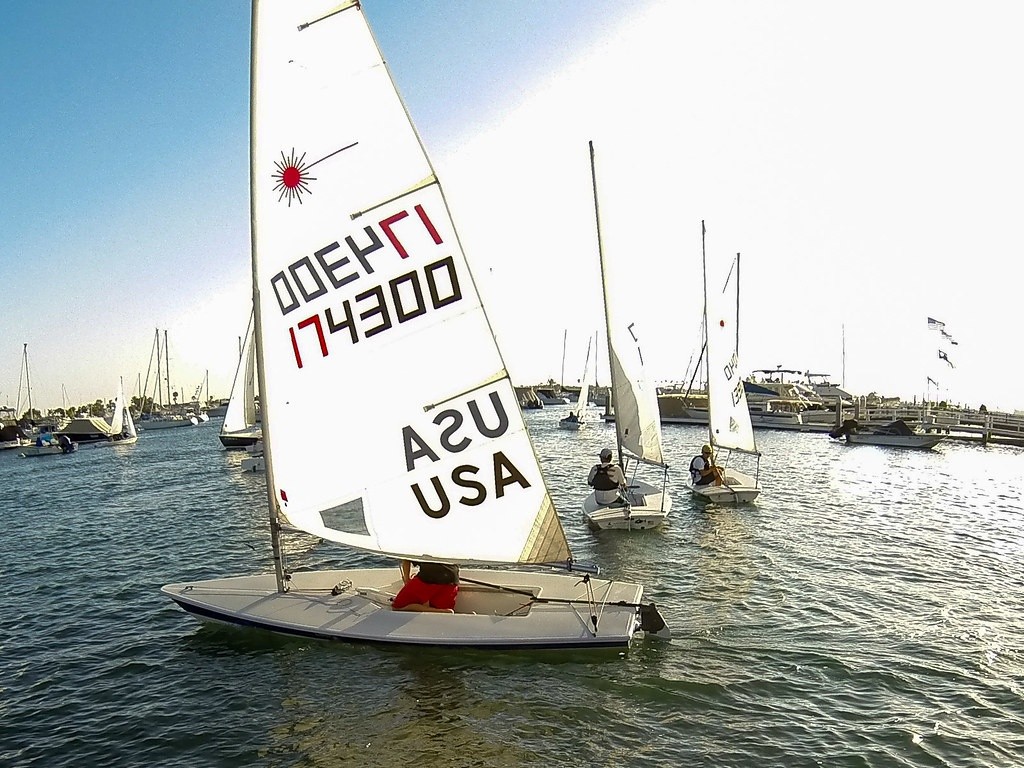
[0,343,55,449]
[676,252,802,425]
[558,336,594,431]
[540,330,571,404]
[581,140,671,531]
[92,375,137,447]
[134,326,261,430]
[219,306,264,449]
[686,220,762,504]
[161,0,674,649]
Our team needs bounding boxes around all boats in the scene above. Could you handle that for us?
[18,429,77,456]
[519,389,545,410]
[843,418,949,449]
[753,363,855,408]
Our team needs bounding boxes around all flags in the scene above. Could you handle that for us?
[939,351,955,369]
[941,331,952,339]
[928,317,945,330]
[928,377,939,390]
[951,339,959,345]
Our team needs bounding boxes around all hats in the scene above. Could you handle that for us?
[597,448,612,459]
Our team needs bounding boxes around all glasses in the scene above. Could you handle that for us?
[705,452,711,454]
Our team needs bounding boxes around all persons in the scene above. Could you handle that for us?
[588,448,629,508]
[688,444,724,487]
[565,411,577,422]
[391,562,460,612]
